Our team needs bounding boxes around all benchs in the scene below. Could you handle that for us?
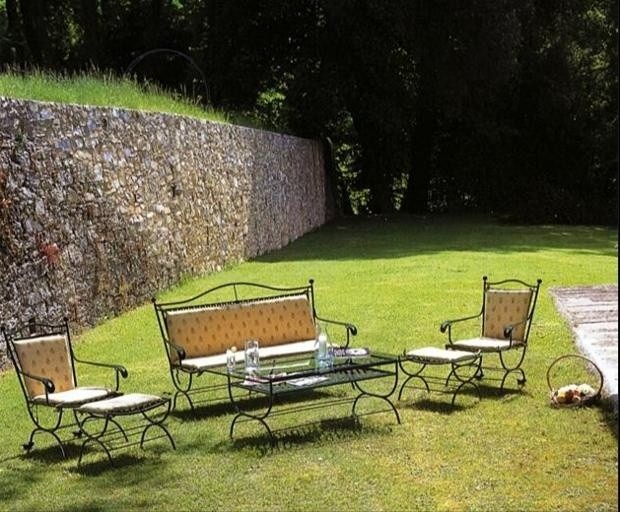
[151,278,357,414]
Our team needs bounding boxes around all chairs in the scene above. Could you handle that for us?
[440,275,542,398]
[2,316,129,459]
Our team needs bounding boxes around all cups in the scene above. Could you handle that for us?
[244,339,260,373]
[226,349,236,372]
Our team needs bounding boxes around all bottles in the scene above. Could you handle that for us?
[314,324,334,366]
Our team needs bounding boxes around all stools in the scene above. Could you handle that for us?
[74,393,176,467]
[398,347,482,411]
